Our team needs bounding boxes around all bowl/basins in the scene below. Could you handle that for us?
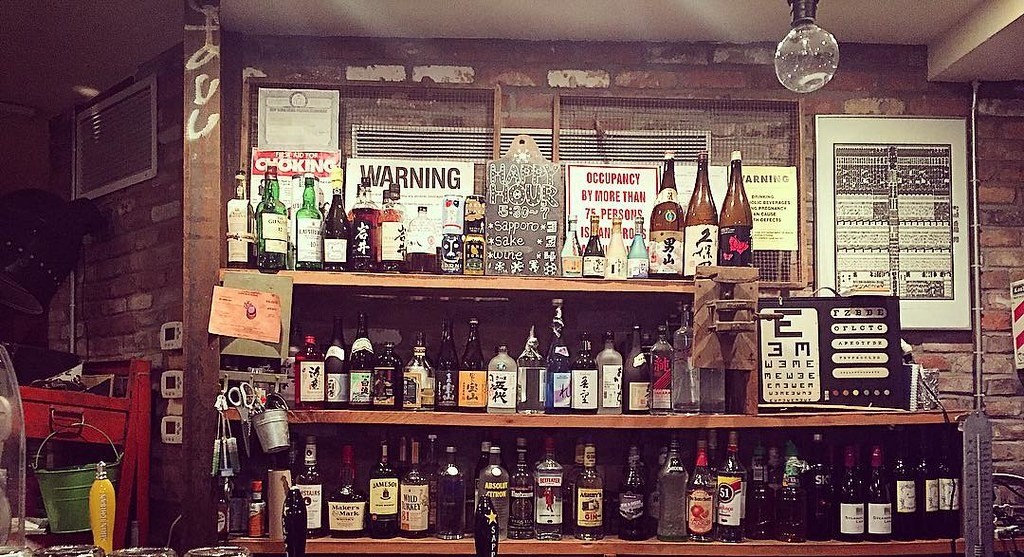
[0,545,251,557]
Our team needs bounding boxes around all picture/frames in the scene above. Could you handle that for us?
[813,114,974,332]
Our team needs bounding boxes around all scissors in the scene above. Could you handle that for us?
[228,382,255,458]
[269,394,300,422]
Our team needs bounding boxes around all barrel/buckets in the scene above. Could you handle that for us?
[251,393,290,453]
[31,422,124,533]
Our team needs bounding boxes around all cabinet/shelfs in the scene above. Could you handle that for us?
[0,359,153,551]
[218,266,1023,557]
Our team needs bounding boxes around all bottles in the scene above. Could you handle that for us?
[226,166,407,275]
[604,217,627,280]
[407,207,437,275]
[627,217,649,279]
[684,150,719,281]
[283,298,728,414]
[717,151,753,266]
[560,214,583,278]
[582,215,606,279]
[89,461,115,555]
[217,436,962,557]
[647,150,684,280]
